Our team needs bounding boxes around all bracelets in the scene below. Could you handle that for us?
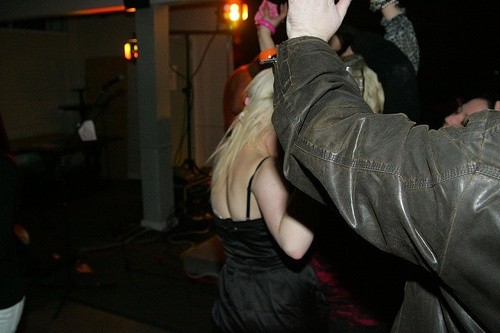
[256,19,275,32]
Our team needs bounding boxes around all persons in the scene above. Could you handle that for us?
[271,0,500,333]
[210,67,332,333]
[223,0,500,138]
